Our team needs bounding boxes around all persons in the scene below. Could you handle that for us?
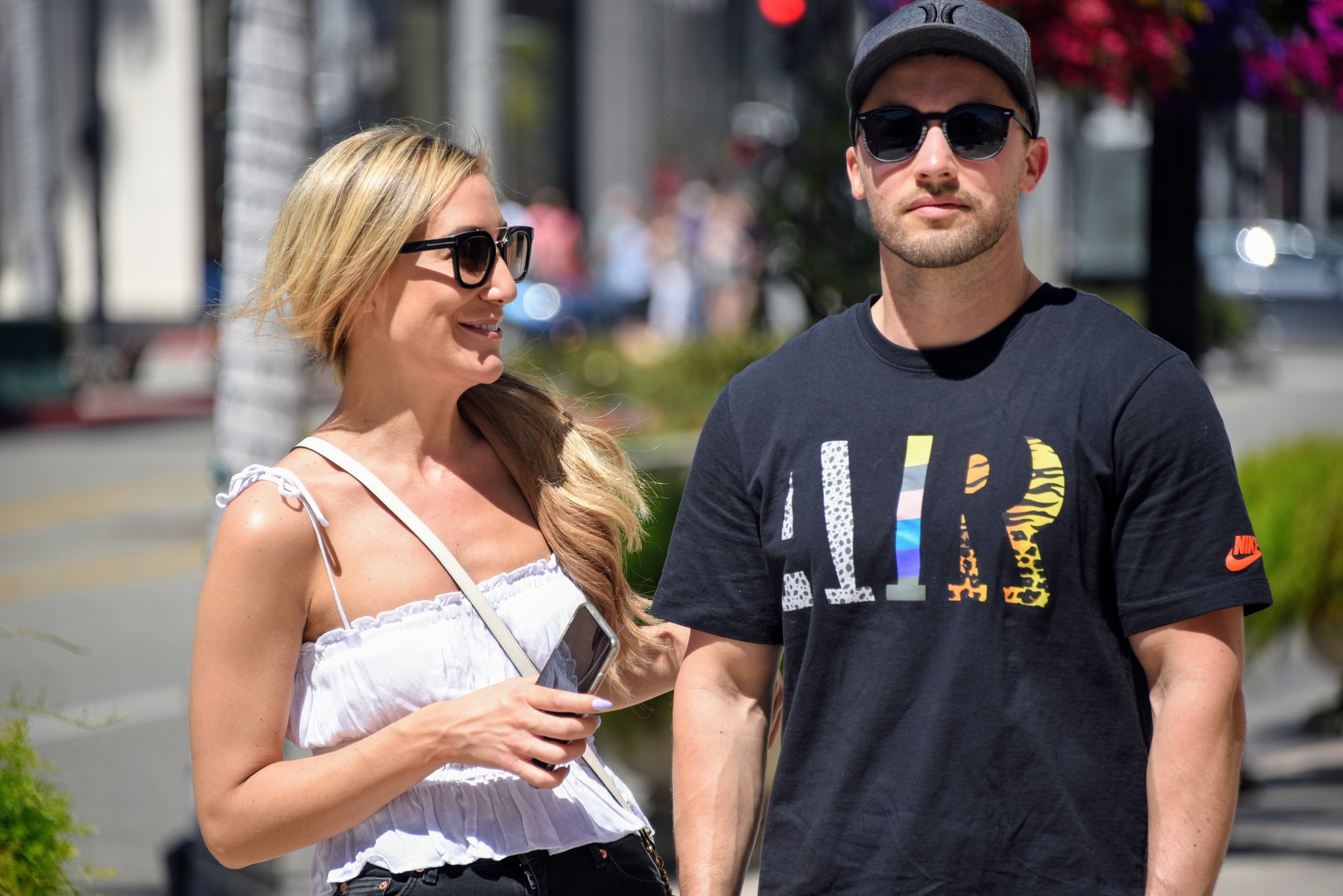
[647,0,1275,896]
[189,125,691,896]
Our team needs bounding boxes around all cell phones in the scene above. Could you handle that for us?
[529,602,621,790]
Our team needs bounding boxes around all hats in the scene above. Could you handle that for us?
[846,0,1040,138]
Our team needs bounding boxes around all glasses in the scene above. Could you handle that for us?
[854,103,1032,163]
[396,226,534,289]
